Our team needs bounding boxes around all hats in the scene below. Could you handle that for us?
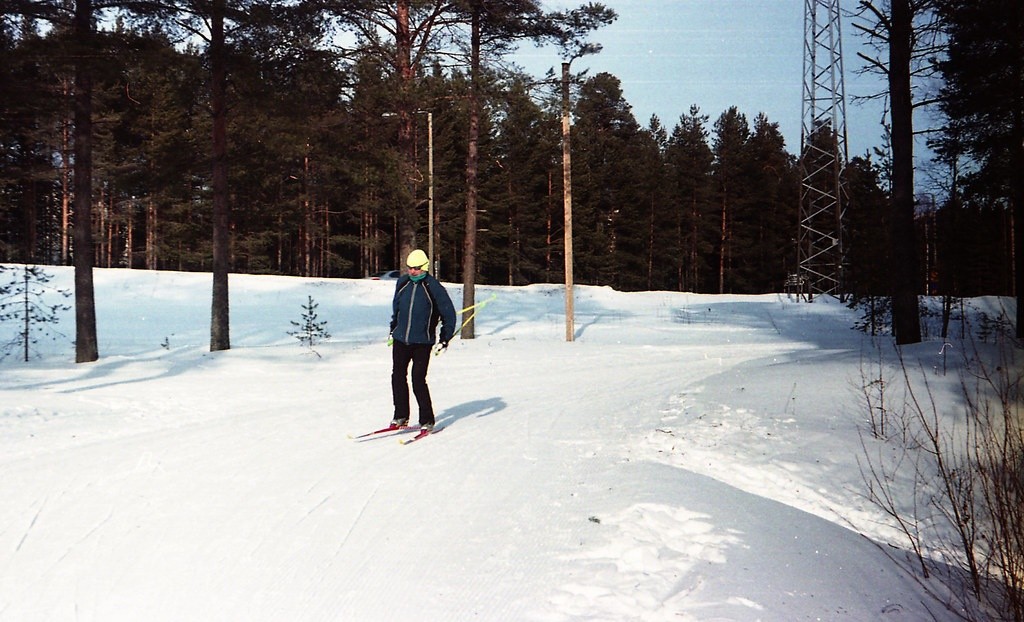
[407,249,429,271]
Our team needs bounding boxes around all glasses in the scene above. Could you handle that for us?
[406,262,428,270]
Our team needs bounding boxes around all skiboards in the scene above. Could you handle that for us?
[345,425,446,445]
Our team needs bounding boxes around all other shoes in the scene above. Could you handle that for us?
[390,417,409,427]
[421,422,434,431]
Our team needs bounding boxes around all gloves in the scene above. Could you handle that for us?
[434,341,448,357]
[387,332,394,346]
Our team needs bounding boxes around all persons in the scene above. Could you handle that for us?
[388,249,456,432]
